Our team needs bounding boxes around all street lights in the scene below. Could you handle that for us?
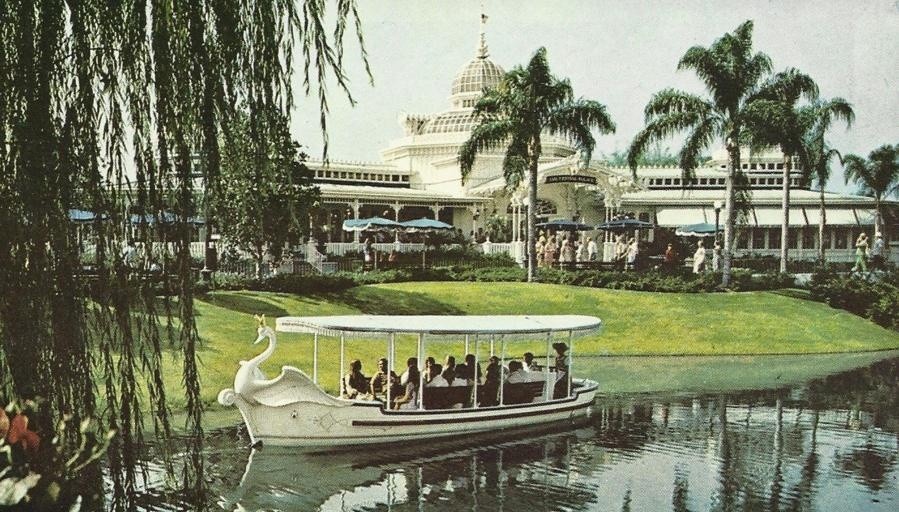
[523,197,530,269]
[712,200,724,242]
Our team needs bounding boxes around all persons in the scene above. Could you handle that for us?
[868,232,883,274]
[534,230,595,271]
[711,241,723,271]
[615,235,648,272]
[362,237,396,272]
[123,239,163,271]
[552,343,572,400]
[666,243,675,260]
[343,227,524,245]
[692,241,706,272]
[344,357,370,401]
[419,459,575,505]
[849,231,870,274]
[371,351,538,408]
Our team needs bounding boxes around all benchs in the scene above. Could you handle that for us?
[503,380,544,404]
[423,387,473,410]
[342,376,401,400]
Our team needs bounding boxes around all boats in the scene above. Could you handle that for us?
[216,313,602,450]
[206,414,597,512]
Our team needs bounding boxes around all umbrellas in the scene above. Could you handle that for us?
[397,217,454,271]
[66,207,112,253]
[597,214,658,270]
[534,217,595,270]
[125,208,205,258]
[342,215,404,268]
[675,219,726,240]
